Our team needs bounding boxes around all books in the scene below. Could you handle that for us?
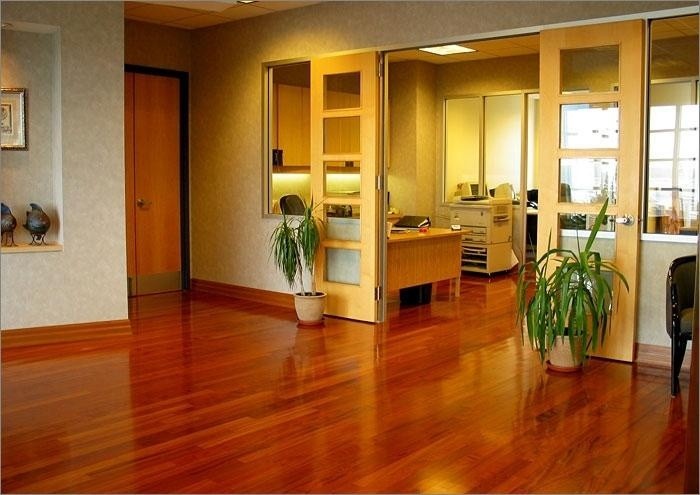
[394,216,431,228]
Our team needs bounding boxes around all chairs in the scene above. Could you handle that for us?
[280,195,306,215]
[666,256,697,398]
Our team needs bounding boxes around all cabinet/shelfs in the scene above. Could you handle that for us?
[448,200,512,277]
[273,83,360,173]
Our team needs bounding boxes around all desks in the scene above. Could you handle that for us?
[387,227,474,297]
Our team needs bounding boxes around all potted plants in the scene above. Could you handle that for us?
[267,192,328,326]
[513,197,629,374]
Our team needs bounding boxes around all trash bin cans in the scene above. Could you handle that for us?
[399,284,432,305]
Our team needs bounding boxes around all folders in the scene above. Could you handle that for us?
[395,215,432,231]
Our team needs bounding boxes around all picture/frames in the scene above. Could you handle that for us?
[0,88,27,150]
